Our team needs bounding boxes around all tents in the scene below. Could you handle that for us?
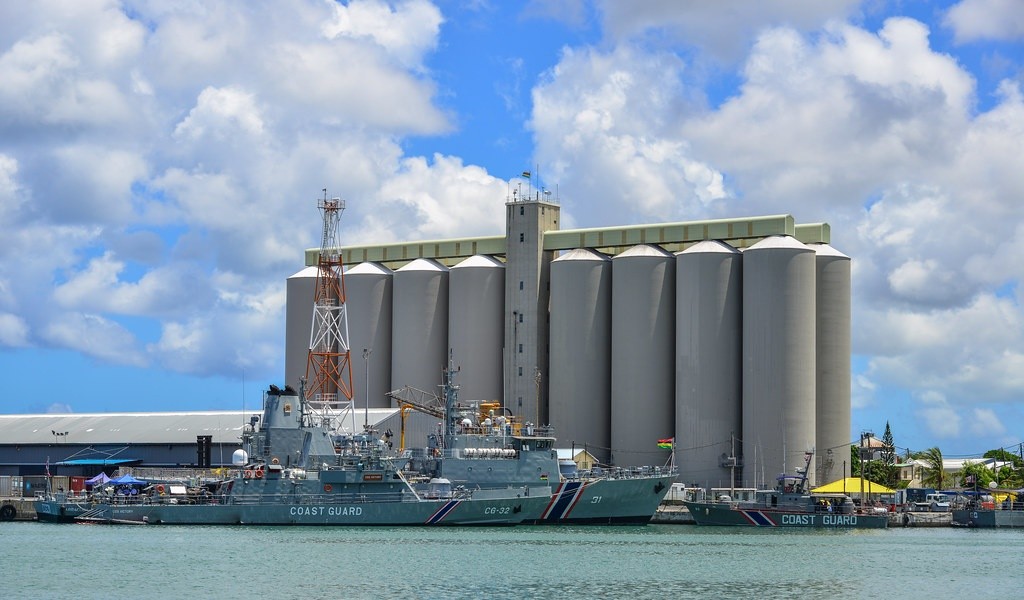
[84,472,112,484]
[810,478,897,508]
[109,474,148,485]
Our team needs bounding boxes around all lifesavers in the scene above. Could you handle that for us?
[156,485,164,494]
[255,470,263,479]
[245,470,252,479]
[324,484,332,492]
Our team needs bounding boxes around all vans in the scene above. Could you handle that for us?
[925,494,953,506]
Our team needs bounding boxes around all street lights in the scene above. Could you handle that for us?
[362,347,373,427]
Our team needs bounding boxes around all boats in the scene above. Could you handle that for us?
[33,348,680,527]
[682,431,890,529]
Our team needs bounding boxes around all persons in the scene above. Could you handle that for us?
[117,488,137,505]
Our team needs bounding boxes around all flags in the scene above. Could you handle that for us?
[656,439,672,449]
[46,461,50,476]
[966,475,973,482]
